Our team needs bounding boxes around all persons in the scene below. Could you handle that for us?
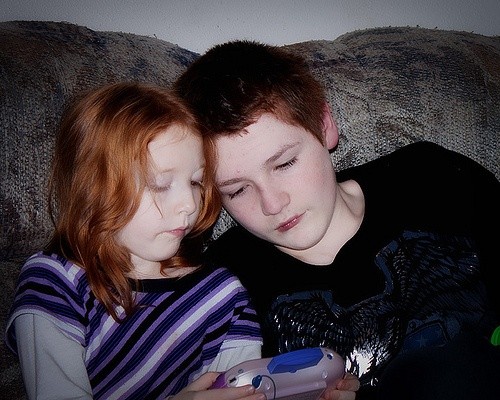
[5,79,360,400]
[172,40,500,400]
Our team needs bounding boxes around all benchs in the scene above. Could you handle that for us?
[0,20,500,400]
